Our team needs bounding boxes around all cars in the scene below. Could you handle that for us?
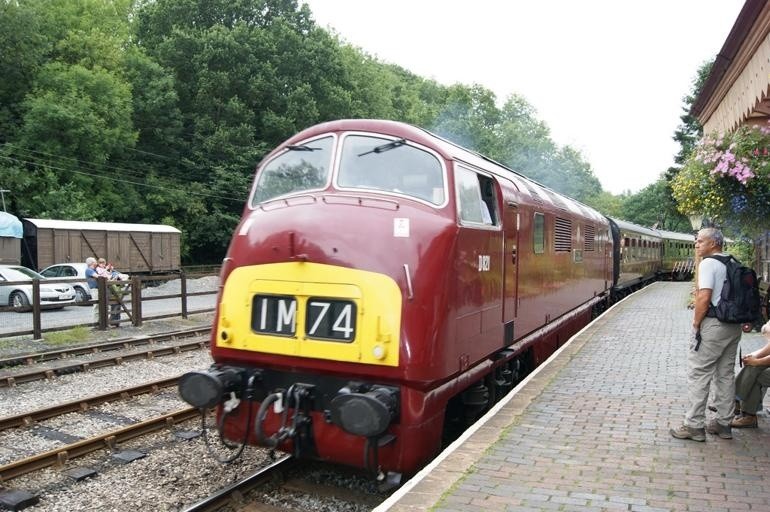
[37,262,132,304]
[0,263,75,312]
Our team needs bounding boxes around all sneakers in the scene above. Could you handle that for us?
[670,402,758,441]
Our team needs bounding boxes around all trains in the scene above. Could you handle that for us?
[0,211,181,276]
[178,119,744,491]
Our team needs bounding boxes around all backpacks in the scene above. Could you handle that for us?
[702,254,760,323]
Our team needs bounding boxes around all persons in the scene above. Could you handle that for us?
[709,317,769,429]
[110,271,127,328]
[671,228,743,443]
[96,258,114,281]
[84,256,100,329]
